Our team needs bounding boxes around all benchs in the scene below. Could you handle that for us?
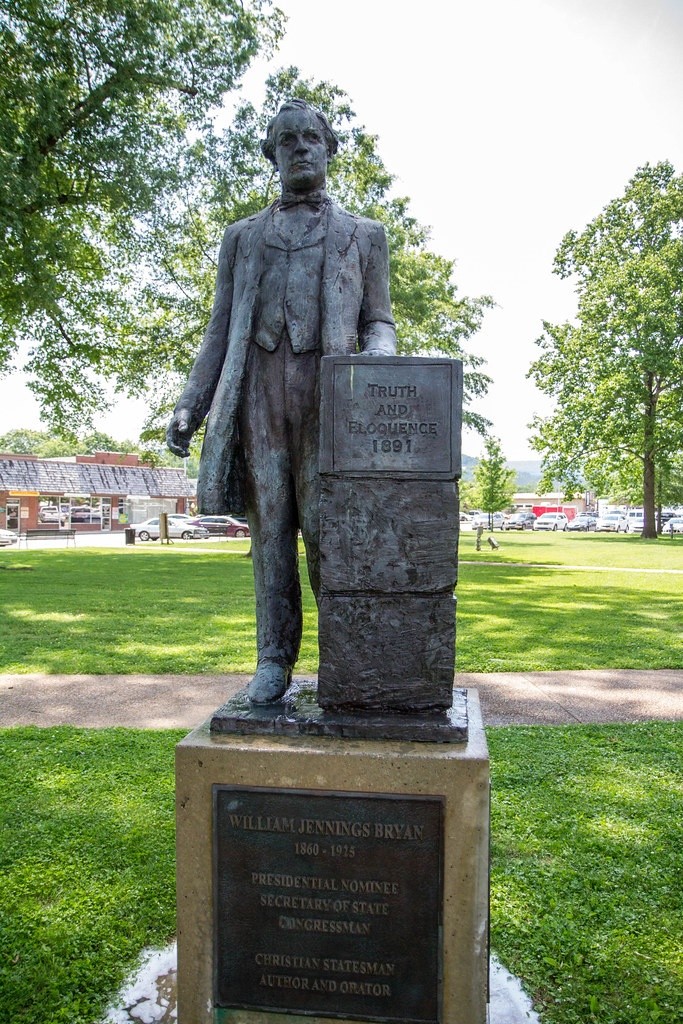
[189,526,229,543]
[16,529,77,550]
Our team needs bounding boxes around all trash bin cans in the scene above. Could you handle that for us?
[125,528,136,545]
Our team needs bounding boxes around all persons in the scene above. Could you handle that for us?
[165,98,397,708]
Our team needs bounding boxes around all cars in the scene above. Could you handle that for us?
[38,497,109,522]
[595,515,631,534]
[472,514,504,531]
[191,516,251,538]
[531,511,568,531]
[0,528,17,547]
[167,514,192,522]
[661,517,683,536]
[654,512,676,527]
[129,517,208,542]
[504,511,537,532]
[565,517,597,532]
[470,509,483,517]
[458,512,472,522]
